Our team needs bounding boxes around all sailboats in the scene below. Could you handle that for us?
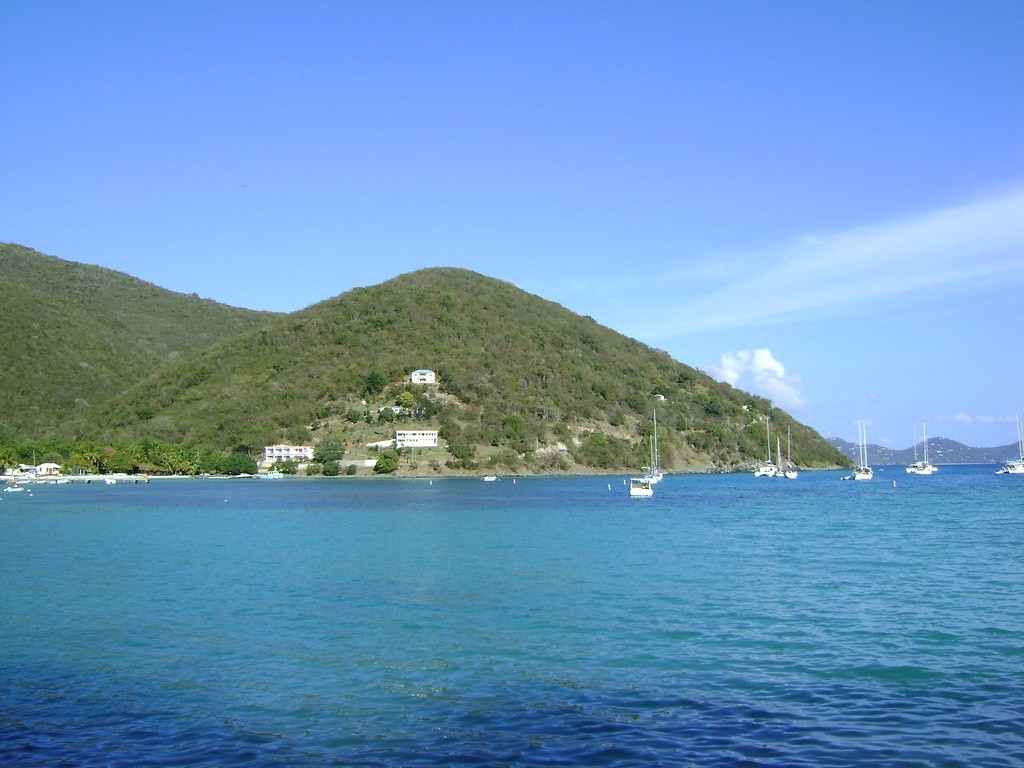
[753,418,798,479]
[993,415,1024,475]
[905,422,938,474]
[629,409,663,484]
[852,419,874,481]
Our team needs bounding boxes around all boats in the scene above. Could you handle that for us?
[630,481,653,496]
